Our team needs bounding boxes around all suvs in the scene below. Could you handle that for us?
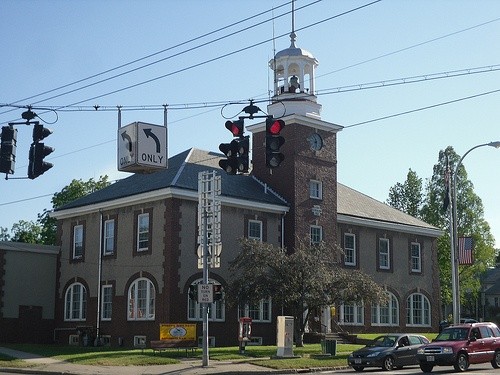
[416,322,500,372]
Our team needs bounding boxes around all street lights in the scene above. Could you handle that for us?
[453,142,500,324]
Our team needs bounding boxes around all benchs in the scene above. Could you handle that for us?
[150,339,200,358]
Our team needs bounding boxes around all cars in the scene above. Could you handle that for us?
[347,334,431,371]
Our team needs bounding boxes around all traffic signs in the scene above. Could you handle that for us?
[118,122,168,170]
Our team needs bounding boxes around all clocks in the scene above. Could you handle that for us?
[309,134,322,151]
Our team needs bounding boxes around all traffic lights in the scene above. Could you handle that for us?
[216,135,250,174]
[224,119,245,137]
[189,284,197,300]
[213,285,222,301]
[28,124,56,179]
[265,117,285,168]
